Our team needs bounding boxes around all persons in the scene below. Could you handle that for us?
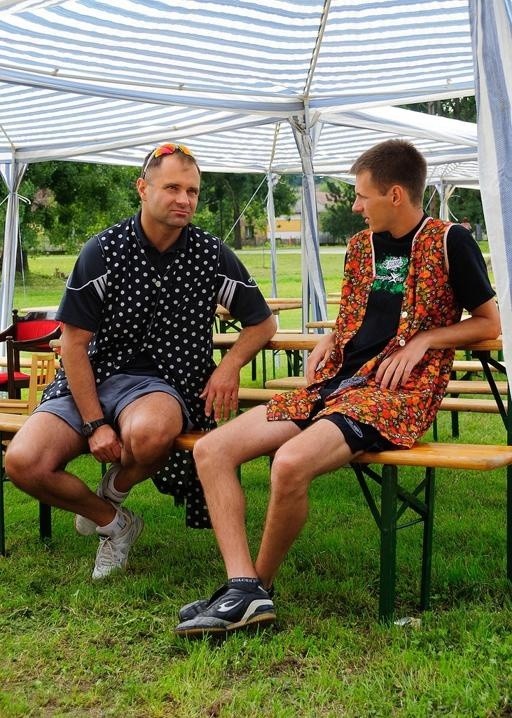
[174,140,502,637]
[4,142,278,580]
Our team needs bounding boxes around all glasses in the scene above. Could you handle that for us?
[144,143,195,176]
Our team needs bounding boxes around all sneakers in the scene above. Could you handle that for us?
[92,506,143,577]
[73,461,129,535]
[175,583,276,637]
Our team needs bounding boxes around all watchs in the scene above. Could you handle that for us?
[81,418,109,436]
